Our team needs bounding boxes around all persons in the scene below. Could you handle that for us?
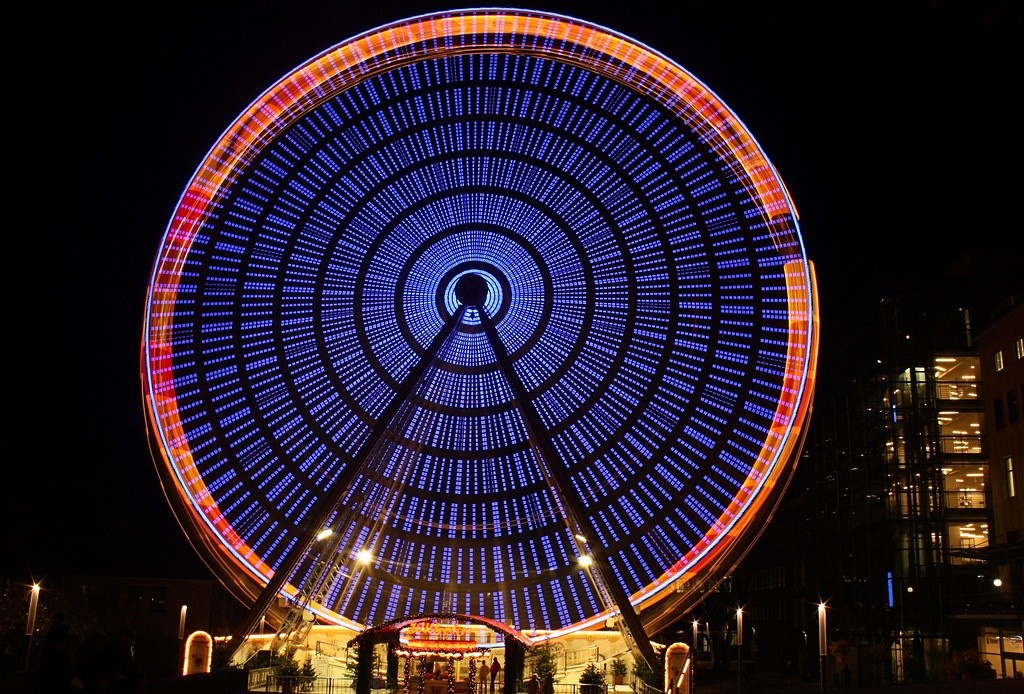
[34,625,136,694]
[844,663,851,688]
[490,657,500,691]
[907,656,929,688]
[527,671,554,694]
[479,660,489,693]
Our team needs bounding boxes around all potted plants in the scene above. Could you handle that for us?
[610,658,627,684]
[276,660,301,694]
